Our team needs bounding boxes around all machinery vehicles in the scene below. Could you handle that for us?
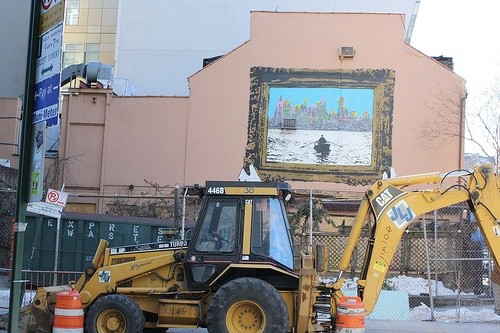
[29,160,500,333]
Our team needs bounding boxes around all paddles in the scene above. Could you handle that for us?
[326,140,342,147]
[300,141,315,148]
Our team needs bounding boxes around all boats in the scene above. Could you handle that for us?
[314,143,330,152]
